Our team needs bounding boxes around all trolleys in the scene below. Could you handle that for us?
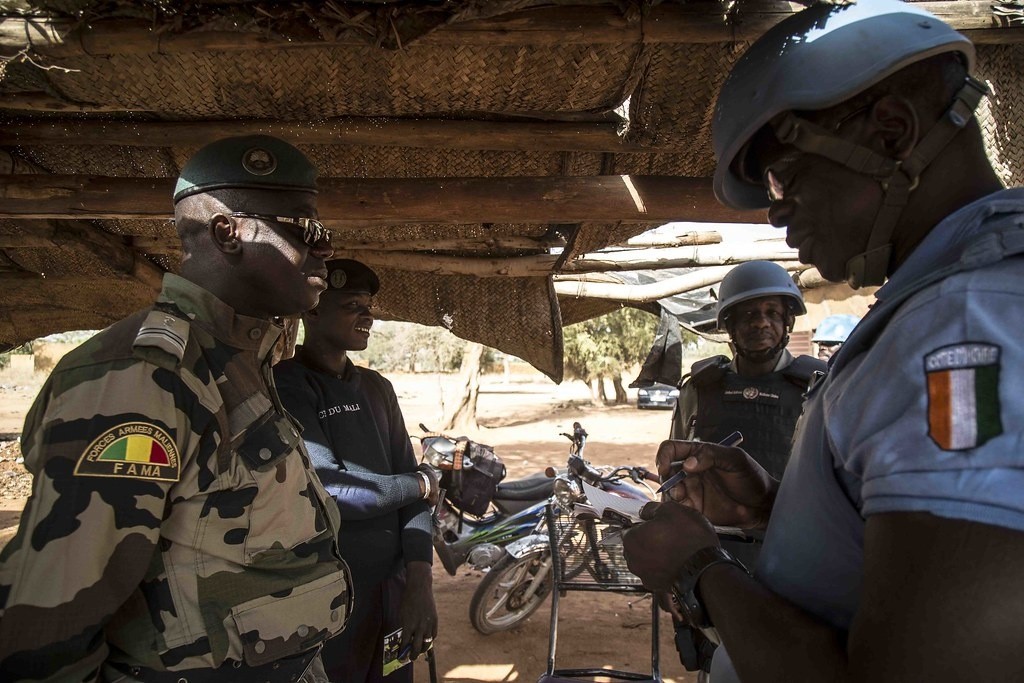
[540,499,667,682]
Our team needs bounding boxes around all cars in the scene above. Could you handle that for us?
[637,383,681,409]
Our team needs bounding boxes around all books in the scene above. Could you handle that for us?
[383,628,434,677]
[573,481,756,544]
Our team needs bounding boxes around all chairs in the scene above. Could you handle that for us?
[538,502,668,683]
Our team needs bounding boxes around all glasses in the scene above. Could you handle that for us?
[206,212,333,251]
[762,84,910,206]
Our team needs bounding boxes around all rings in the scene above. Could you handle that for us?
[423,637,432,642]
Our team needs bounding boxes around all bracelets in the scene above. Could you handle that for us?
[416,470,430,499]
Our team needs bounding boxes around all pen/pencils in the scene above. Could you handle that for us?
[655,430,744,494]
[398,646,412,662]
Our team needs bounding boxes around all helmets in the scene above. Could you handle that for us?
[711,0,976,212]
[716,261,807,331]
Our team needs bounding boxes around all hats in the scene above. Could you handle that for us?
[324,258,380,297]
[173,134,319,208]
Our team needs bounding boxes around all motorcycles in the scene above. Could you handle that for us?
[469,421,672,634]
[410,424,617,586]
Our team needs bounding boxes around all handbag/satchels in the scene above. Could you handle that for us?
[420,431,506,517]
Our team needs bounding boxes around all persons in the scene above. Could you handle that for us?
[670,259,826,683]
[0,137,355,683]
[269,258,440,683]
[811,313,860,362]
[622,2,1024,682]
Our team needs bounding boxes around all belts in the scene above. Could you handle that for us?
[105,640,327,683]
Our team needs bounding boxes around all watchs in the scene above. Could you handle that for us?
[668,546,755,631]
